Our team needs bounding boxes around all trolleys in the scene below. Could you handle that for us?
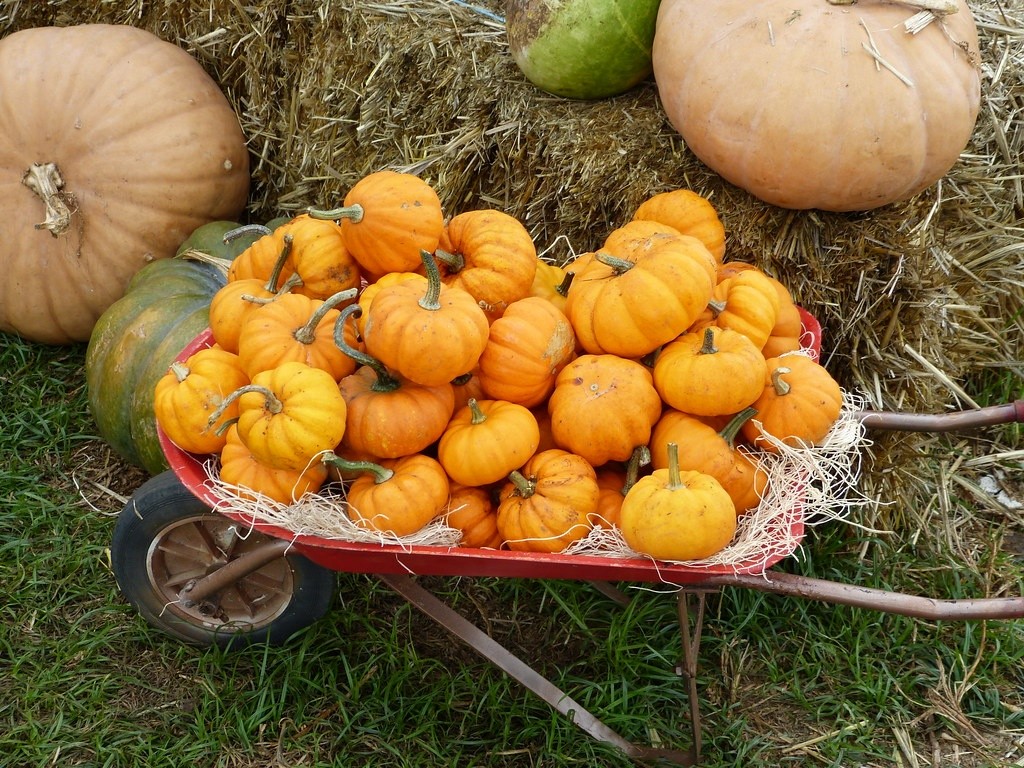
[110,304,1024,656]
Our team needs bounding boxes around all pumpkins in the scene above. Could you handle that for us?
[505,0,661,98]
[85,172,843,561]
[652,1,981,213]
[0,24,248,344]
